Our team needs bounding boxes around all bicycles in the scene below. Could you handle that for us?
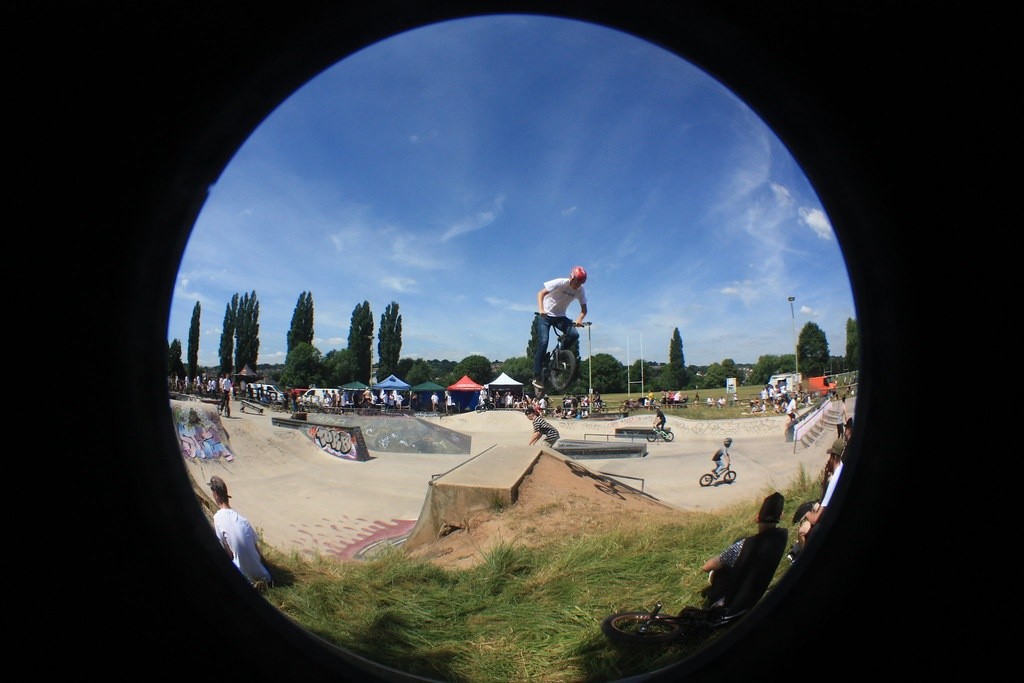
[646,423,674,442]
[217,390,231,418]
[475,398,496,414]
[532,311,586,392]
[699,464,737,487]
[601,592,750,649]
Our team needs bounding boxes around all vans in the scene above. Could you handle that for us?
[290,389,308,398]
[246,383,284,406]
[302,389,355,408]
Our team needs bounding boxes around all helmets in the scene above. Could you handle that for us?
[571,266,586,284]
[724,437,732,445]
[525,408,533,415]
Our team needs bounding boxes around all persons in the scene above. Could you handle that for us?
[479,386,488,409]
[430,392,439,412]
[701,491,787,606]
[751,383,814,413]
[786,394,800,421]
[445,390,452,415]
[844,416,853,440]
[844,373,856,393]
[624,399,641,410]
[524,406,561,449]
[168,373,418,410]
[797,438,845,549]
[694,394,699,403]
[531,266,588,389]
[504,391,513,407]
[707,395,714,406]
[716,396,726,409]
[733,393,737,400]
[494,390,500,408]
[645,390,660,409]
[837,394,848,437]
[514,390,602,418]
[653,405,666,431]
[206,475,271,584]
[219,374,231,417]
[661,390,688,403]
[712,438,733,477]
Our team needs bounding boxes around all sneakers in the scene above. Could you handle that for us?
[531,378,544,388]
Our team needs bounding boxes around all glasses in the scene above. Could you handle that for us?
[572,277,582,287]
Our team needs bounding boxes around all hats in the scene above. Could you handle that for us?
[827,439,845,456]
[759,492,785,523]
[207,475,228,496]
[793,499,815,523]
[844,418,856,427]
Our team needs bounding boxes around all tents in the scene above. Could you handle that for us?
[337,372,523,413]
